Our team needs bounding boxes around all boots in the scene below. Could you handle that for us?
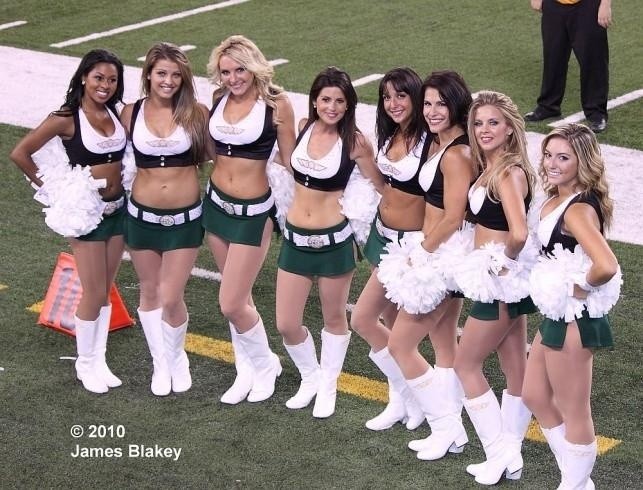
[365,347,426,432]
[74,303,122,393]
[281,326,352,420]
[541,423,599,490]
[406,364,469,460]
[461,388,532,485]
[136,307,192,397]
[221,307,283,404]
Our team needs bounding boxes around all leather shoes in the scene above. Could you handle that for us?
[523,105,562,121]
[588,113,608,132]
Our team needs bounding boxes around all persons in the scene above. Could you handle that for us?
[272,65,383,420]
[115,41,217,398]
[9,48,126,396]
[525,121,621,490]
[450,88,535,485]
[386,71,480,462]
[347,68,438,432]
[520,0,614,135]
[199,34,297,407]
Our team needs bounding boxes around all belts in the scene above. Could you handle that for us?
[205,181,274,217]
[377,216,403,243]
[127,200,204,226]
[283,222,352,247]
[101,197,124,216]
